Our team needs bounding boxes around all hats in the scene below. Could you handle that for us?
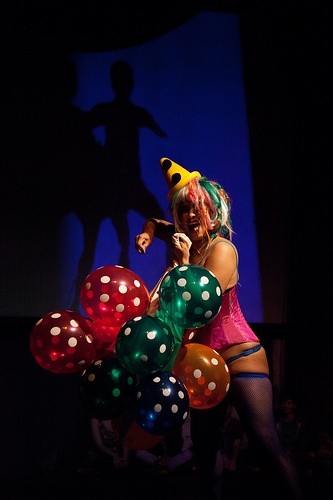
[159,157,201,201]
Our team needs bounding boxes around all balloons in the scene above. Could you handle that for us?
[31,265,230,451]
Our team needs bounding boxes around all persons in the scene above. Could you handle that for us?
[134,157,301,492]
[88,321,333,500]
